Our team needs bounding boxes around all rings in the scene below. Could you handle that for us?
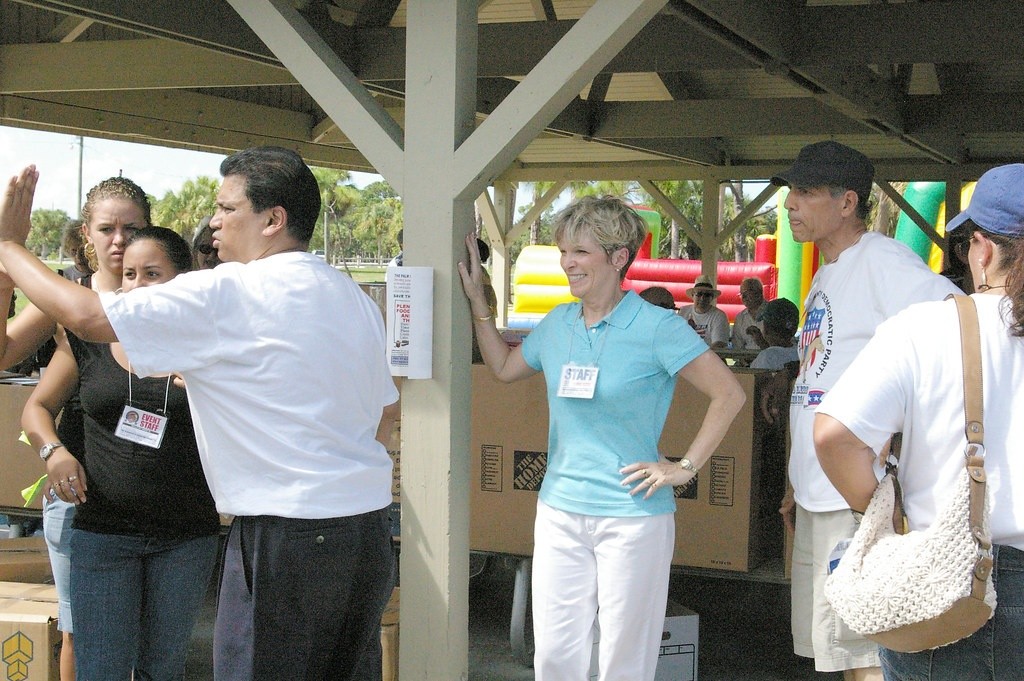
[69,477,77,482]
[55,484,59,487]
[643,469,648,477]
[653,484,657,487]
[60,480,68,484]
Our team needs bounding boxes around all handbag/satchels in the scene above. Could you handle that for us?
[822,457,997,655]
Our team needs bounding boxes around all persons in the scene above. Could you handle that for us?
[0,179,152,681]
[473,238,497,361]
[61,220,85,279]
[193,216,223,269]
[385,229,402,281]
[767,142,1024,680]
[643,275,800,368]
[0,145,402,681]
[21,226,219,681]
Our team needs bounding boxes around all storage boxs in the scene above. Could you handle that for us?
[590,595,698,681]
[383,363,795,580]
[380,585,403,681]
[0,371,67,681]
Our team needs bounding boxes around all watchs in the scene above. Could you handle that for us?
[40,443,63,462]
[678,459,699,474]
[851,510,863,522]
[457,195,745,681]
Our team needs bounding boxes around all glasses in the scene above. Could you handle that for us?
[198,244,219,257]
[694,292,713,298]
[955,233,999,264]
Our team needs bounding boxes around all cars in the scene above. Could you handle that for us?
[311,249,324,260]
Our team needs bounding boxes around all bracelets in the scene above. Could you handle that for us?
[472,308,494,321]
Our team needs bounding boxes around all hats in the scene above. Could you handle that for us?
[770,141,874,201]
[686,275,721,300]
[944,164,1024,238]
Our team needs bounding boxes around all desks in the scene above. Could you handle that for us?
[468,550,791,671]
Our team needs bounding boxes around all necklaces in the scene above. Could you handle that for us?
[977,284,1005,293]
